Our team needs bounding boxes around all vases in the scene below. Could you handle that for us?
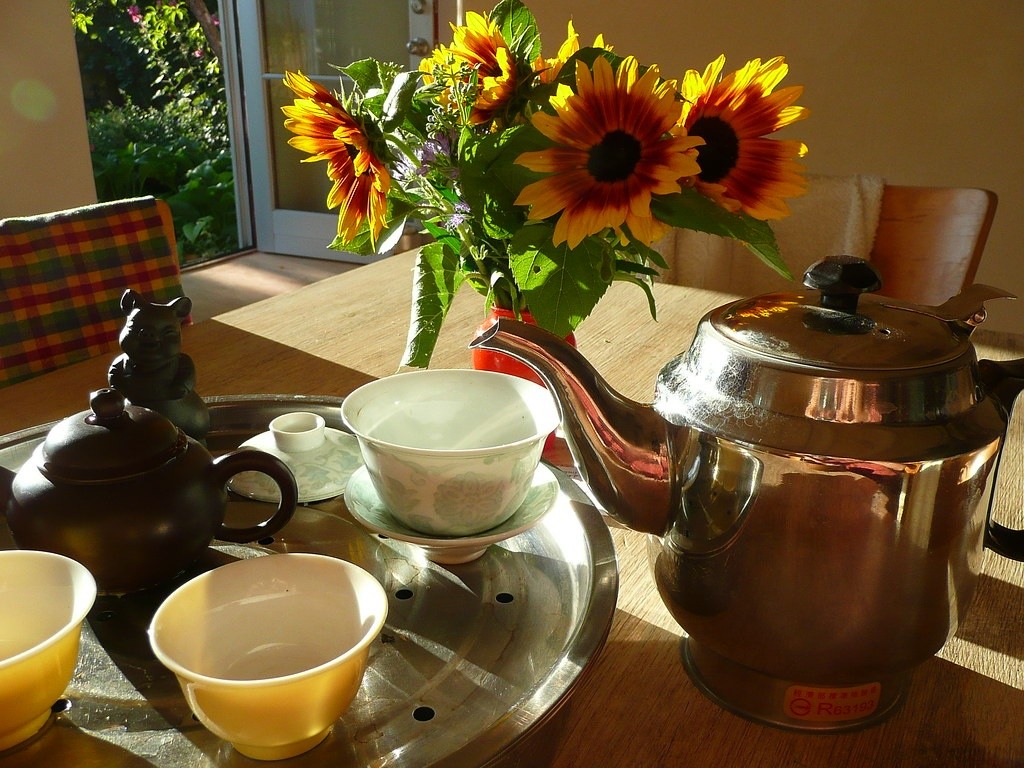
[469,305,577,454]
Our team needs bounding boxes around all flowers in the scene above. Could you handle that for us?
[273,0,826,345]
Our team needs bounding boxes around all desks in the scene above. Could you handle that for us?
[0,233,1024,768]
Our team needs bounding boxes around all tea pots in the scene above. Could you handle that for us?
[466,255,1024,735]
[0,388,297,598]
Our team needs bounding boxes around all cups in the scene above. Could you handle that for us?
[0,549,96,752]
[340,369,560,538]
[147,554,388,763]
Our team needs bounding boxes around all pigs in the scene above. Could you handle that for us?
[107,288,209,448]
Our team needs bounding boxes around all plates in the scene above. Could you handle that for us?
[344,465,559,565]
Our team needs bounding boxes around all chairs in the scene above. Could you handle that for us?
[638,172,1001,328]
[0,194,192,391]
